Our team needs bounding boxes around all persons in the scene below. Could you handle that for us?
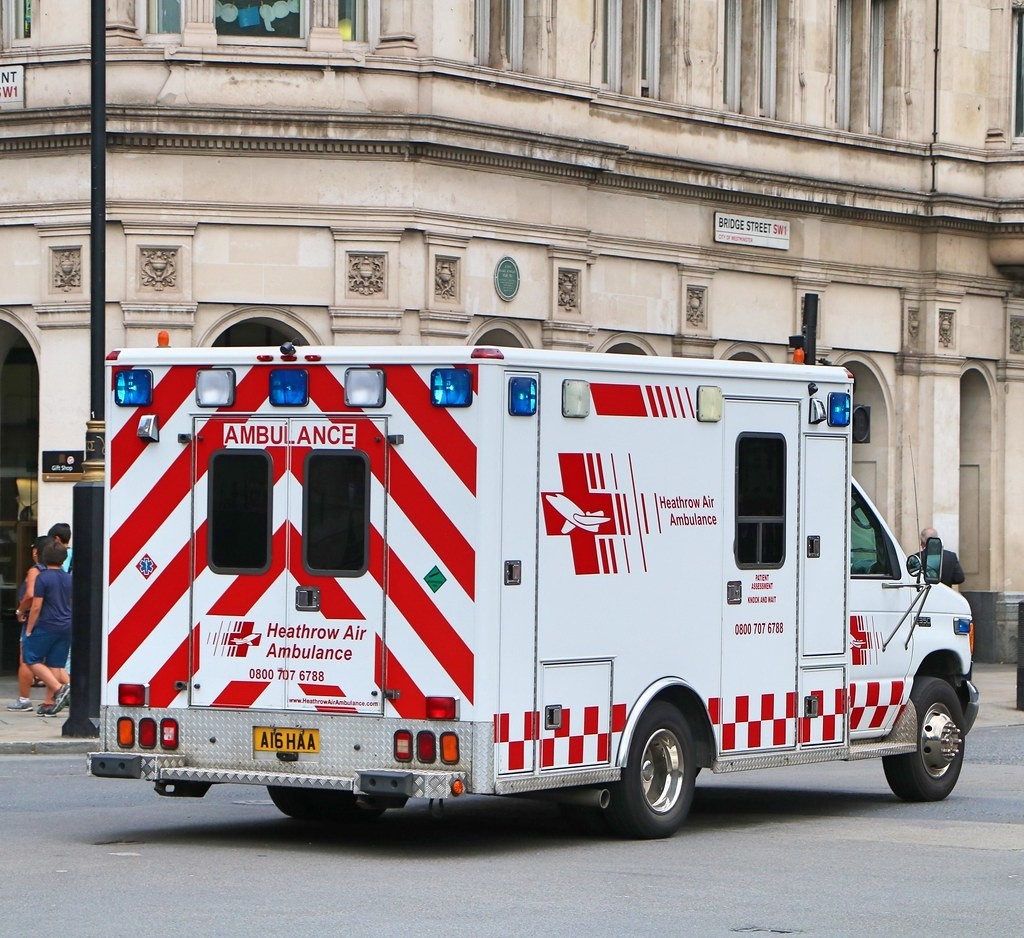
[914,528,965,589]
[22,543,72,717]
[7,522,73,712]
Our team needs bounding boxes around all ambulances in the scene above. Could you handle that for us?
[87,330,980,841]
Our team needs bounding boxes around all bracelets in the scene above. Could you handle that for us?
[16,610,23,616]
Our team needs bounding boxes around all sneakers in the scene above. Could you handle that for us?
[50,682,71,714]
[7,697,34,711]
[36,703,56,716]
